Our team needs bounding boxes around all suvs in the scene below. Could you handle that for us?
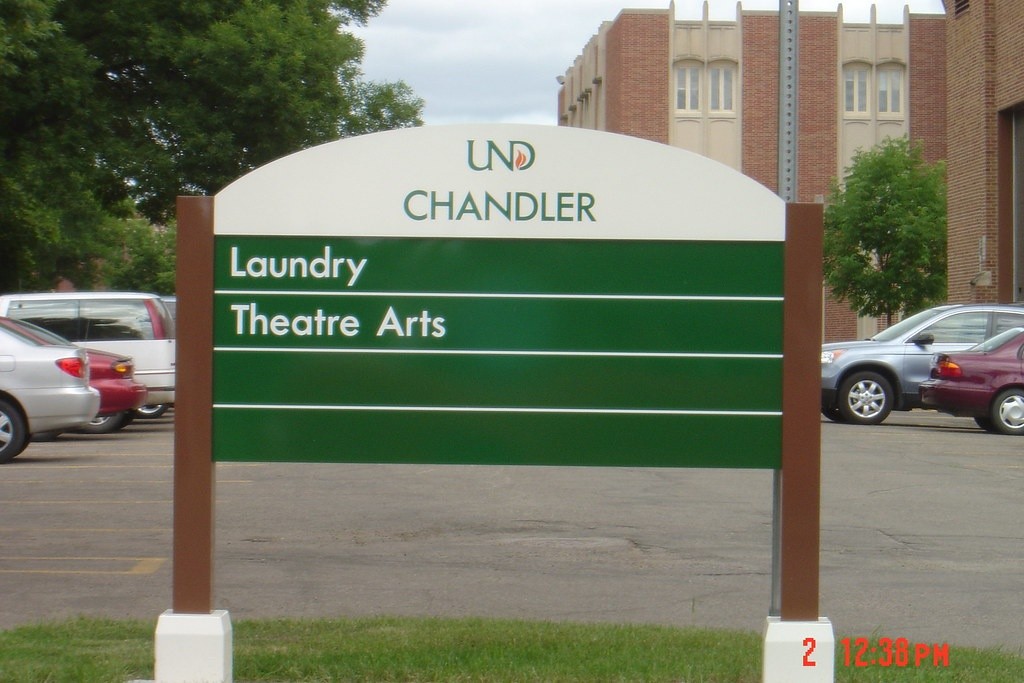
[820,302,1023,425]
[0,292,176,434]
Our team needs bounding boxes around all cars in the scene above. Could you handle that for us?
[0,327,101,465]
[0,315,150,443]
[917,324,1024,434]
